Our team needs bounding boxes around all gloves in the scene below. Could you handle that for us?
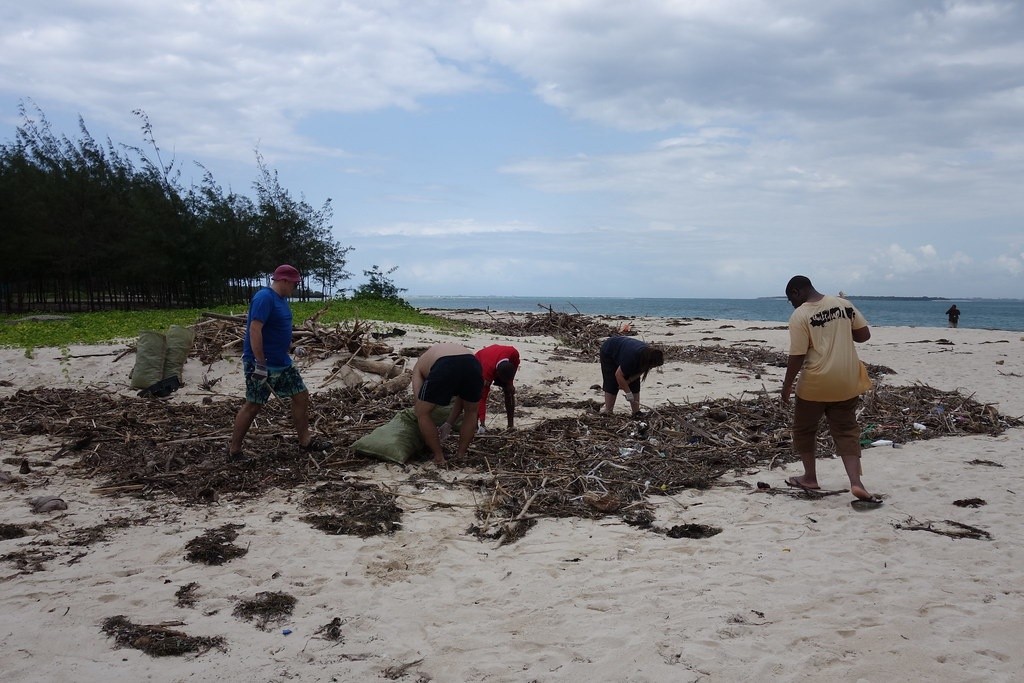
[626,392,634,402]
[290,346,311,357]
[251,365,268,385]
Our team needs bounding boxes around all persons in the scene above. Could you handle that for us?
[413,343,484,465]
[229,264,332,461]
[946,304,960,328]
[474,344,520,432]
[782,275,883,503]
[600,336,664,414]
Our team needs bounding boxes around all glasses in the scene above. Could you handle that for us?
[786,293,792,304]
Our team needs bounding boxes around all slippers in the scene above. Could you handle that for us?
[851,496,883,504]
[785,476,820,490]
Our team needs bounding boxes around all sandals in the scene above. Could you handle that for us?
[226,452,256,470]
[299,439,332,455]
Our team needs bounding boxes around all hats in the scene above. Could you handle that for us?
[272,265,301,282]
